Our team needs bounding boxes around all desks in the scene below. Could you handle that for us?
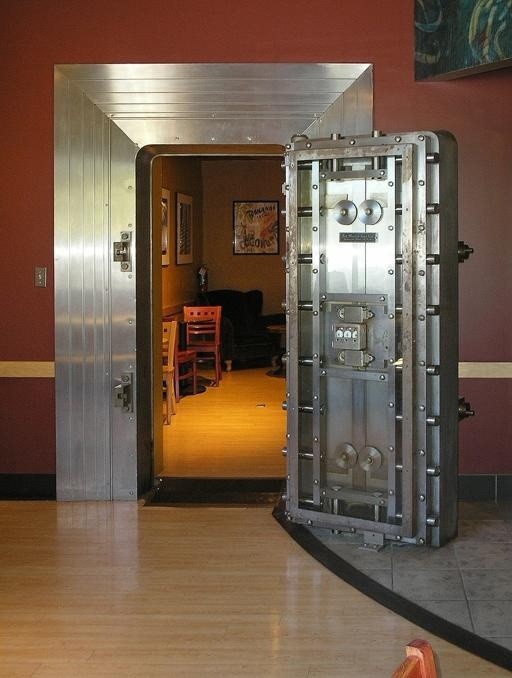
[264,323,286,379]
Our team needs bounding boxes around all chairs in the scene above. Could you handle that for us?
[162,304,223,424]
[196,290,283,372]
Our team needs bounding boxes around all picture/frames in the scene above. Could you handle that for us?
[159,187,195,267]
[231,200,280,258]
